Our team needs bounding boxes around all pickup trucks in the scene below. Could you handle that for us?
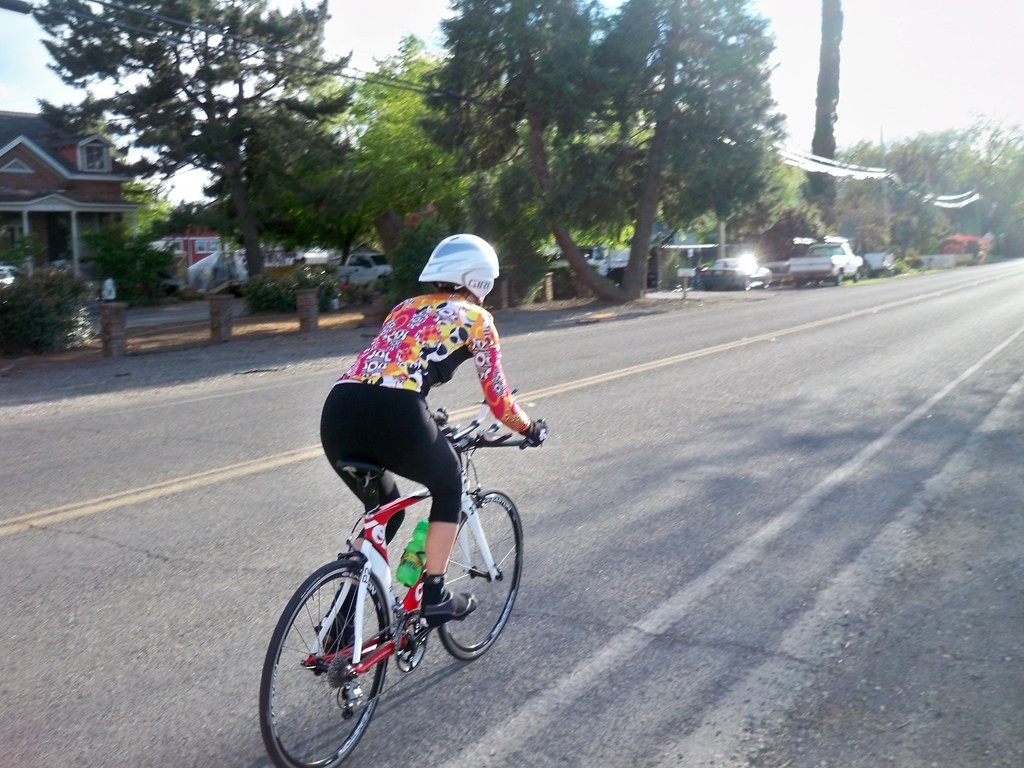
[788,243,864,285]
[539,243,631,284]
[334,252,395,287]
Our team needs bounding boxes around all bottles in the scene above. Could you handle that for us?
[395,519,429,588]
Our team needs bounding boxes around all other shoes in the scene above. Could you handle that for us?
[423,589,478,620]
[315,619,354,654]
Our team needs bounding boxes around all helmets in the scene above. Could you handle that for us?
[418,233,500,302]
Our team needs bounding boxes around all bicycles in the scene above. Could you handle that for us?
[257,389,546,768]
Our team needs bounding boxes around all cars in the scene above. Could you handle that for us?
[1,265,22,289]
[699,258,773,291]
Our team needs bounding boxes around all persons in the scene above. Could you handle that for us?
[319,233,545,649]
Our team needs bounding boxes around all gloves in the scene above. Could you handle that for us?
[520,420,548,447]
[428,410,448,427]
